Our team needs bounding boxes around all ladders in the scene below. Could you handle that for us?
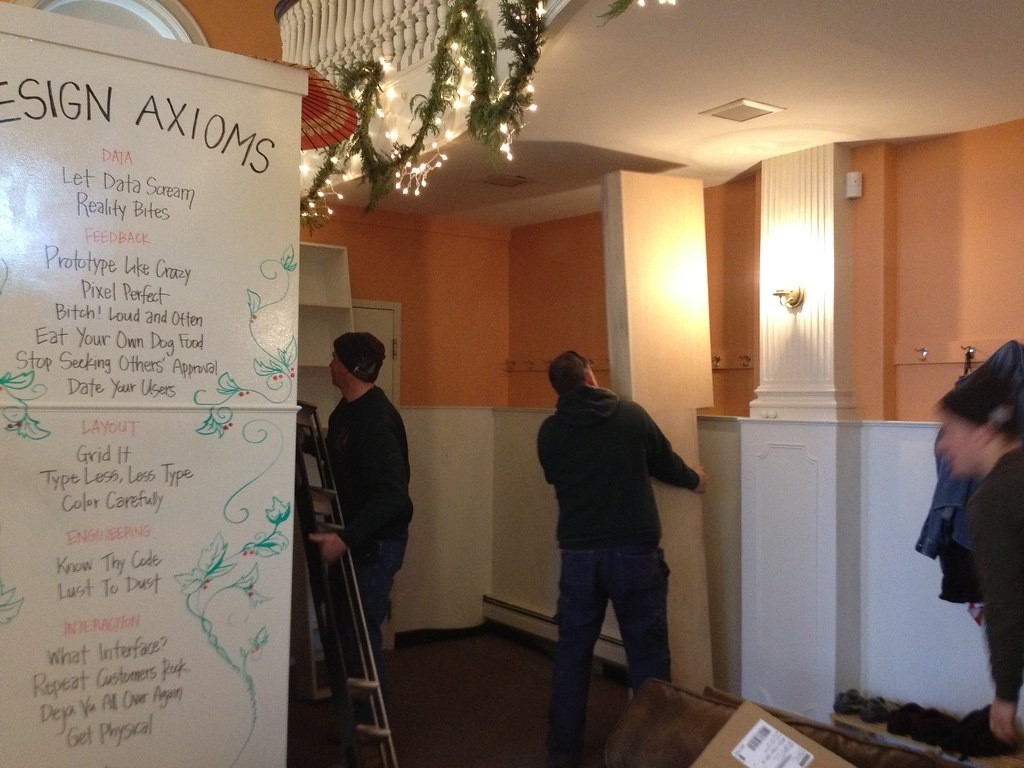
[295,398,399,768]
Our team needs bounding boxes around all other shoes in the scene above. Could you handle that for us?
[834,689,1017,756]
[545,731,582,768]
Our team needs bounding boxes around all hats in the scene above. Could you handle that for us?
[940,366,1024,430]
[333,333,385,383]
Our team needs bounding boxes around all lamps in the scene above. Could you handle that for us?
[768,267,801,308]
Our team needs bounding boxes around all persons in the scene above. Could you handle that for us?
[935,341,1024,746]
[297,331,414,745]
[538,349,709,768]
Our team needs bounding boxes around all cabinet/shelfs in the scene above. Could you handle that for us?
[299,241,356,429]
